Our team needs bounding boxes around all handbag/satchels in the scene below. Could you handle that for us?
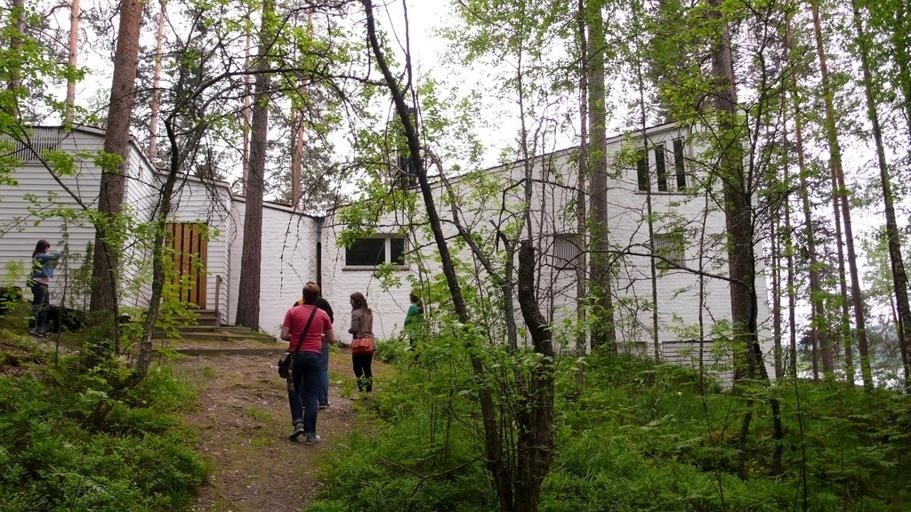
[351,338,377,355]
[278,351,296,379]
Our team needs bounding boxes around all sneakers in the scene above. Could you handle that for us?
[319,401,330,409]
[289,424,305,439]
[305,434,322,446]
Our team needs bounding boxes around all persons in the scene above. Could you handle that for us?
[347,292,376,394]
[281,284,334,447]
[293,281,334,409]
[29,240,65,337]
[404,291,424,366]
